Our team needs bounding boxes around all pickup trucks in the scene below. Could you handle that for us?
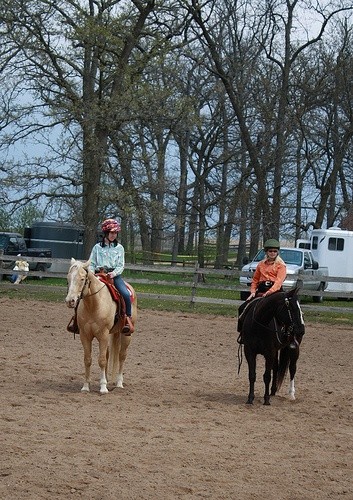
[239,247,329,302]
[0,231,53,283]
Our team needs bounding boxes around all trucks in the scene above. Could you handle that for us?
[295,226,353,301]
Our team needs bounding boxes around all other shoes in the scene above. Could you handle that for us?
[237,335,241,343]
[69,326,79,334]
[123,326,131,336]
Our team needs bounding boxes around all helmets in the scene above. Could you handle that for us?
[264,239,280,249]
[102,219,121,232]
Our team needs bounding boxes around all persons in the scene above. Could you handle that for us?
[237,239,287,330]
[67,218,131,337]
[7,254,30,285]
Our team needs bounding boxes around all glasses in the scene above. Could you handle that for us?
[269,250,277,252]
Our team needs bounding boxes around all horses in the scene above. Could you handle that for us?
[232,283,307,407]
[63,257,139,396]
